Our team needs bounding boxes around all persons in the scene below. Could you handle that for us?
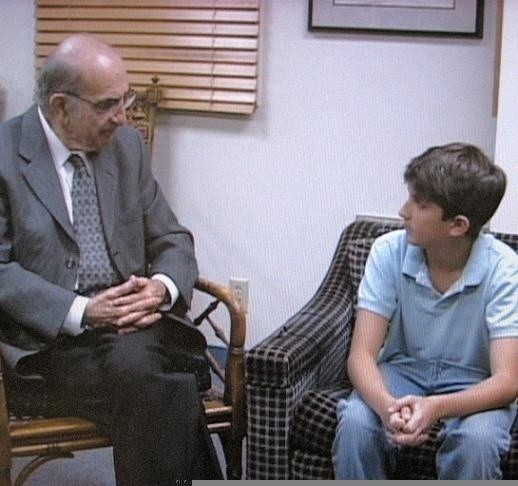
[328,141,518,481]
[0,33,227,486]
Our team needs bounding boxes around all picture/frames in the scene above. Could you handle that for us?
[308,0,485,40]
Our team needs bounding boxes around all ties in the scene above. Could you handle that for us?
[67,152,111,297]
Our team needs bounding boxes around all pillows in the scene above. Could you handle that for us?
[347,238,377,311]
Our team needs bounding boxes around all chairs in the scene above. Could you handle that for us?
[247,215,518,480]
[0,72,248,484]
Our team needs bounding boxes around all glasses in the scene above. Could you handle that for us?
[65,87,138,114]
